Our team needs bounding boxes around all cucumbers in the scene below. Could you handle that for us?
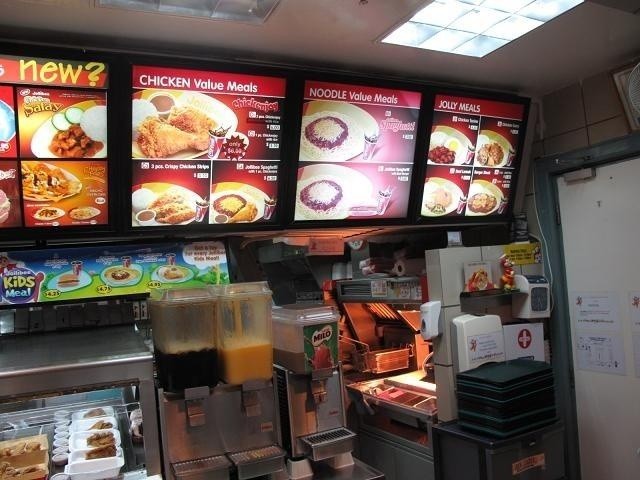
[52,107,85,131]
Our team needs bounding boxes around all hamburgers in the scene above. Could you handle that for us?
[57,275,80,288]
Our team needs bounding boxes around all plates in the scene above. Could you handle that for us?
[30,98,108,158]
[466,178,506,216]
[131,182,204,226]
[421,175,466,216]
[427,125,472,168]
[474,130,518,168]
[32,206,66,221]
[131,90,239,159]
[210,181,271,224]
[21,162,83,202]
[295,164,373,220]
[67,207,101,221]
[297,99,379,163]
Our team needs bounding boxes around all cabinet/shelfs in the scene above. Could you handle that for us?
[0,326,162,480]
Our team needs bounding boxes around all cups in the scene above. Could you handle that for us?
[497,196,509,215]
[506,148,516,167]
[376,191,390,215]
[122,255,131,268]
[194,200,209,223]
[263,199,277,219]
[456,196,467,215]
[206,129,227,160]
[362,137,377,161]
[71,260,83,277]
[167,254,176,266]
[464,144,475,165]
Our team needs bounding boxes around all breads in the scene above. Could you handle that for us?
[87,420,112,429]
[0,441,43,476]
[86,433,116,447]
[83,407,106,417]
[86,443,117,459]
